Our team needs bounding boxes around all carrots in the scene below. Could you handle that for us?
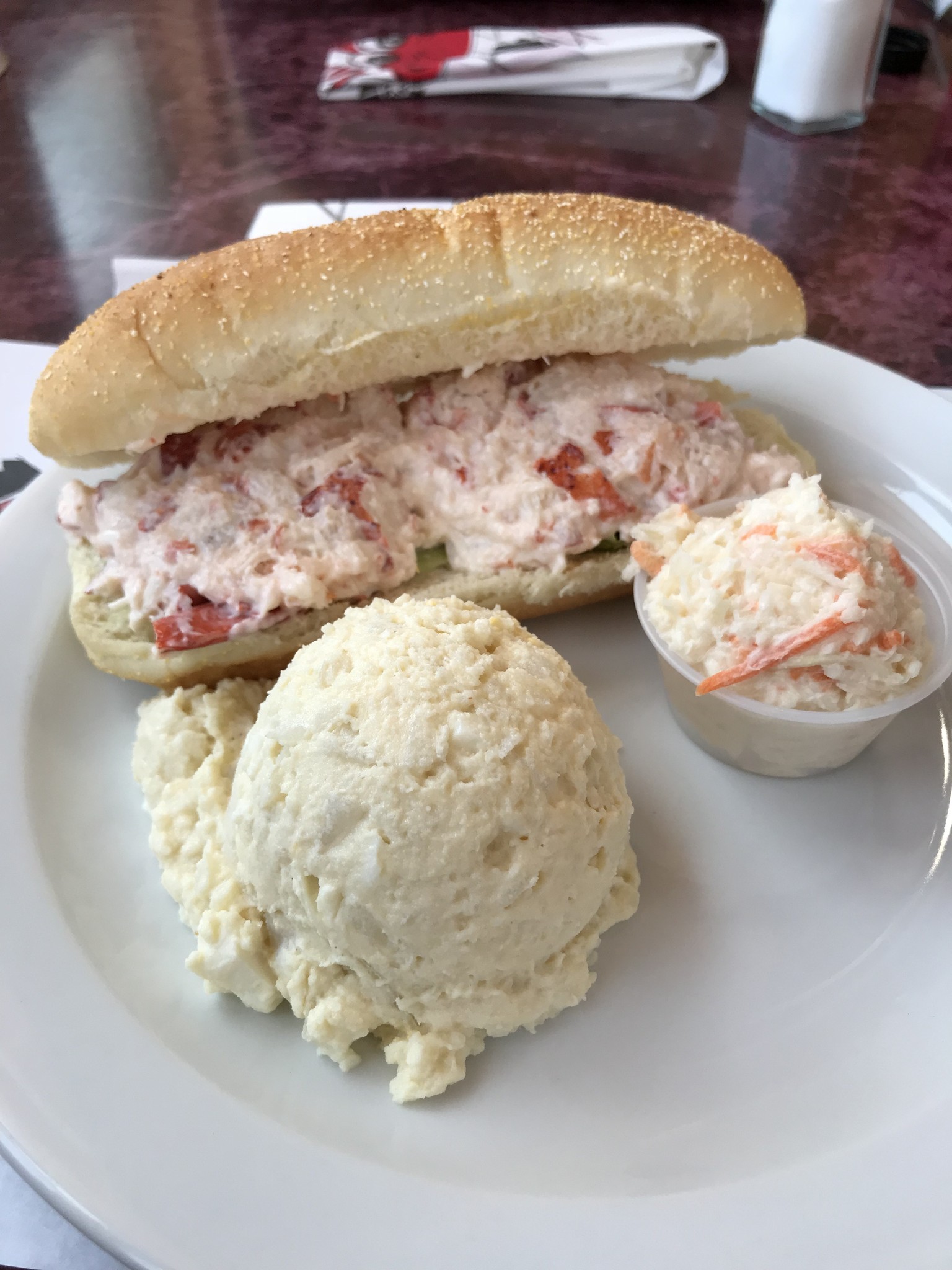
[631,505,917,695]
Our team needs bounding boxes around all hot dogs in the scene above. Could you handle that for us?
[29,191,818,689]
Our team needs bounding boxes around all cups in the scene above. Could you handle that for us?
[750,0,891,132]
[729,113,865,274]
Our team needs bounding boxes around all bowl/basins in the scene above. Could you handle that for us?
[632,492,952,778]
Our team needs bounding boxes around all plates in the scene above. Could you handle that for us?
[0,334,951,1270]
